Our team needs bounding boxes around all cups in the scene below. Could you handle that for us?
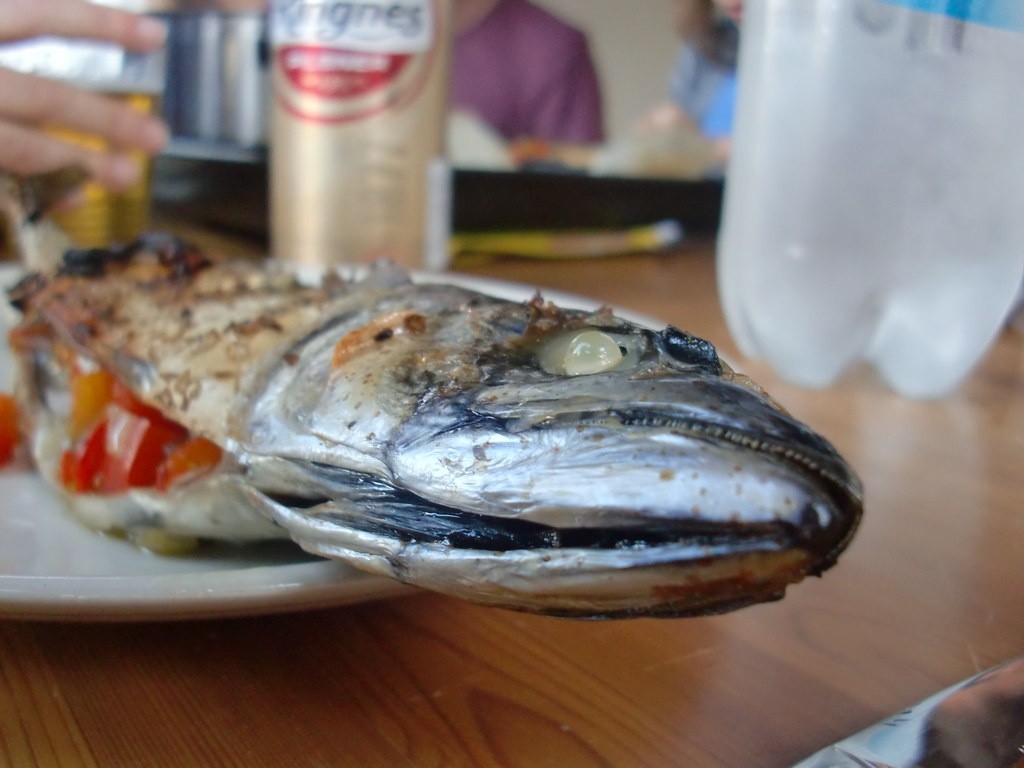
[0,20,168,275]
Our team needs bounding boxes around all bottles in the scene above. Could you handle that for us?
[270,0,449,273]
[717,0,1024,397]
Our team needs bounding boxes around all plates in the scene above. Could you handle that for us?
[1,273,739,623]
[792,665,996,768]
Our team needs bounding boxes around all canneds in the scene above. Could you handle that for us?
[266,1,453,271]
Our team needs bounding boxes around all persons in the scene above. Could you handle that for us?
[445,2,607,153]
[0,1,175,197]
[666,0,741,150]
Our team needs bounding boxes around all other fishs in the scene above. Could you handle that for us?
[1,182,867,624]
